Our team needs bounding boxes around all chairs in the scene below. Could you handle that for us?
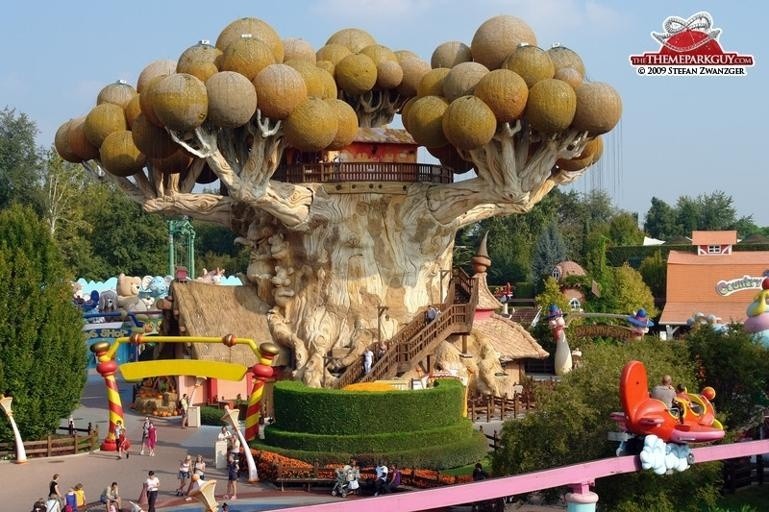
[208,400,248,420]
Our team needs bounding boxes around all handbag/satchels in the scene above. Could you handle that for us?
[367,479,376,491]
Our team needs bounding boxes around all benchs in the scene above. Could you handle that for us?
[85,501,104,509]
[276,476,416,492]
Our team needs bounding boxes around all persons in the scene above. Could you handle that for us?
[426,304,435,320]
[379,341,387,357]
[114,416,158,459]
[473,463,488,481]
[31,474,122,512]
[677,384,691,406]
[143,470,161,512]
[435,306,441,314]
[348,459,400,495]
[652,375,676,408]
[175,393,240,512]
[363,347,374,373]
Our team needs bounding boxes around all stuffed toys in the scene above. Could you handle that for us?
[73,267,227,321]
